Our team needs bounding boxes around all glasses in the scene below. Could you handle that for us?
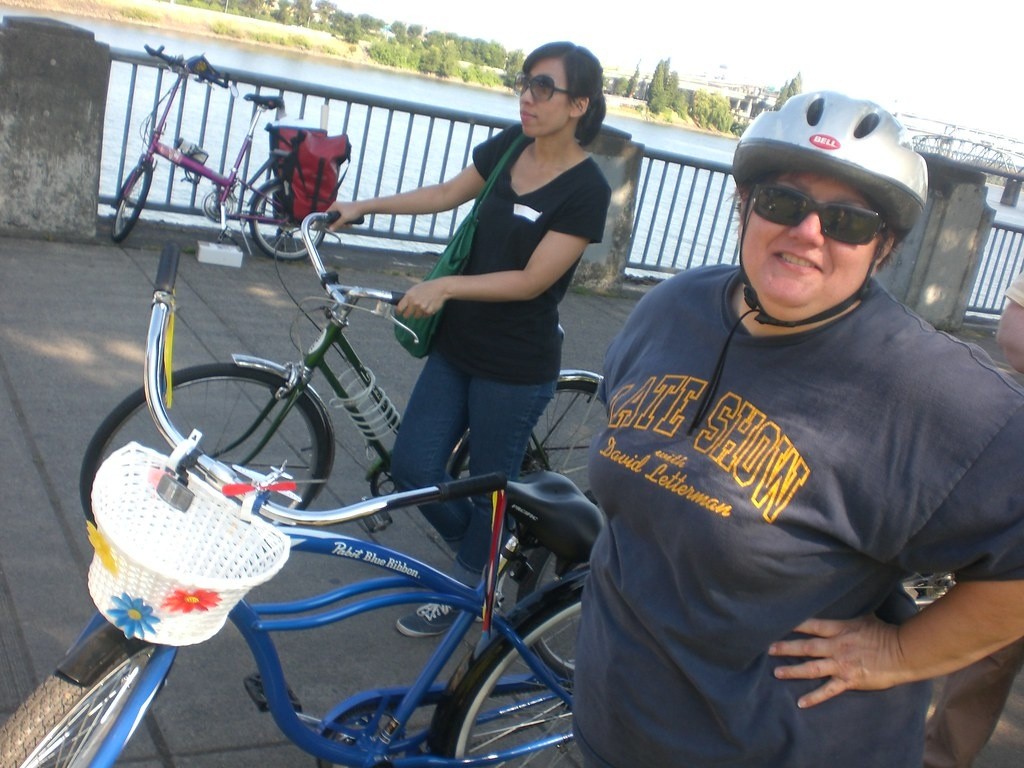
[512,71,572,103]
[751,183,885,245]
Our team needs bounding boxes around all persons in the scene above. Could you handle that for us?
[327,41,613,640]
[923,270,1024,768]
[572,91,1024,768]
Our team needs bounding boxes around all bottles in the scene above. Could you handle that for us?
[174,138,208,165]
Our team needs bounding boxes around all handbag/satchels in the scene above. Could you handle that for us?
[288,127,350,224]
[273,121,327,222]
[393,130,525,359]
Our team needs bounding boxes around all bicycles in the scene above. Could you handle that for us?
[1,238,605,767]
[79,209,606,586]
[110,43,330,261]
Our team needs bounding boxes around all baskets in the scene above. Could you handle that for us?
[87,440,291,647]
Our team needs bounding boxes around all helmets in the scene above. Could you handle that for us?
[732,89,929,245]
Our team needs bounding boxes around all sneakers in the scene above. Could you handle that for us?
[396,603,461,638]
